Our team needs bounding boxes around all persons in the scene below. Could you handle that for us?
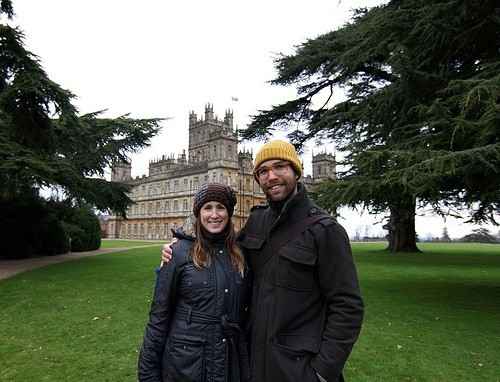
[139,185,251,382]
[159,140,365,382]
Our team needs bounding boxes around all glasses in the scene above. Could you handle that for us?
[253,160,291,180]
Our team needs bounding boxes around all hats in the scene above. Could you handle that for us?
[193,182,237,218]
[252,140,303,184]
